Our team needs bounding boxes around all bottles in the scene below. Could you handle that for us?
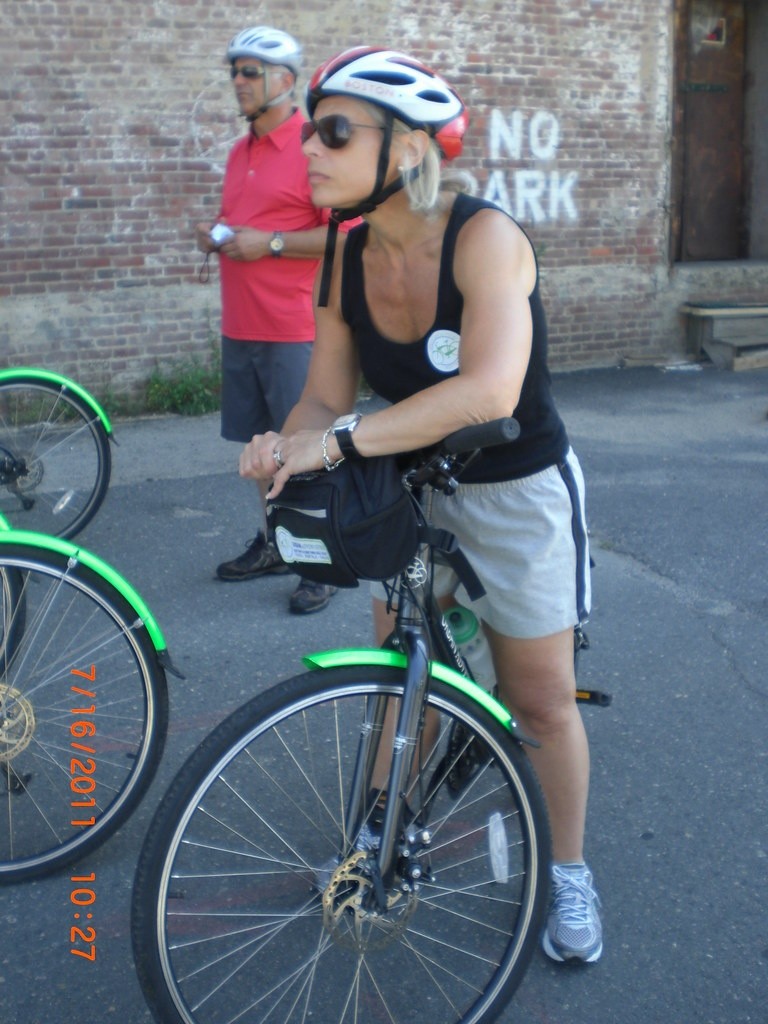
[442,607,497,691]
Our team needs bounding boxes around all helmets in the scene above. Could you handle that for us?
[307,44,471,160]
[225,25,301,81]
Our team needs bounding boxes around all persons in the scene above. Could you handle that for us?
[238,46,602,968]
[198,27,340,612]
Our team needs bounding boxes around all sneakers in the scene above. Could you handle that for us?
[310,824,416,902]
[215,527,293,580]
[288,574,338,613]
[543,863,604,964]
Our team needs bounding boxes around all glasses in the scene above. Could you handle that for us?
[231,65,288,79]
[301,114,404,149]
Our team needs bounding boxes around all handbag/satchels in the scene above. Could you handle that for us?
[264,451,418,588]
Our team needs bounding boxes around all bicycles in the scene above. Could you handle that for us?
[0,368,121,549]
[128,416,613,1024]
[0,513,187,886]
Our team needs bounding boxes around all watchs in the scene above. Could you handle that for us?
[272,231,283,256]
[332,413,365,462]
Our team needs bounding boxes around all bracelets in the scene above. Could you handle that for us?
[274,451,283,468]
[322,427,345,472]
